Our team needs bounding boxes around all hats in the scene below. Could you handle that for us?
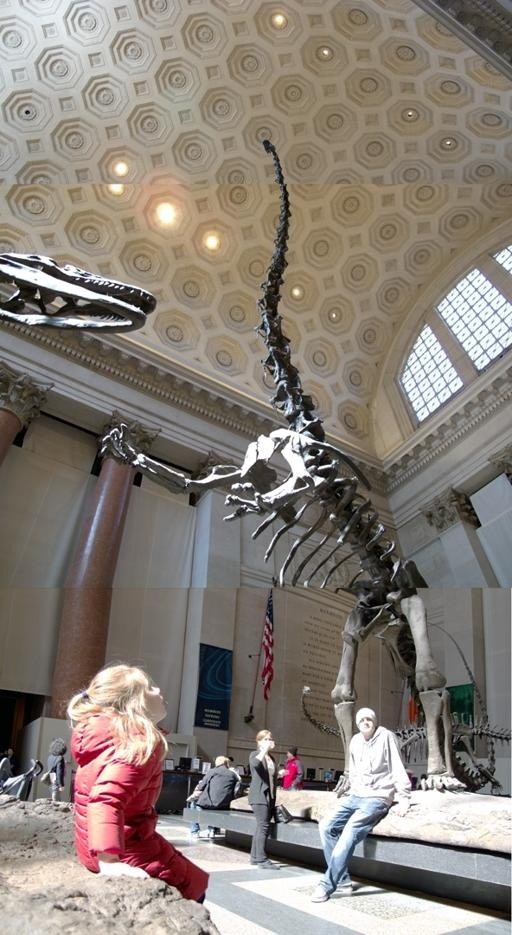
[289,746,297,755]
[355,708,378,725]
[215,756,231,766]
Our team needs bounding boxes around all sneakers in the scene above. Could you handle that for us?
[312,885,329,902]
[207,832,214,838]
[334,886,353,893]
[252,859,280,870]
[192,832,200,838]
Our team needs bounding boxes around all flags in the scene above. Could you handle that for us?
[261,589,274,700]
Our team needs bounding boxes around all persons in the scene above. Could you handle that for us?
[190,753,237,839]
[247,728,289,871]
[281,746,305,790]
[65,661,211,905]
[0,748,16,792]
[227,755,242,796]
[47,738,68,800]
[308,707,414,903]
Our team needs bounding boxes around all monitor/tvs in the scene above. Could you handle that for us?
[307,768,315,780]
[179,757,192,770]
[334,771,344,782]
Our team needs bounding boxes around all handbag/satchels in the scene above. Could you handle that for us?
[41,771,52,785]
[232,780,244,799]
[186,791,201,802]
[273,804,292,823]
